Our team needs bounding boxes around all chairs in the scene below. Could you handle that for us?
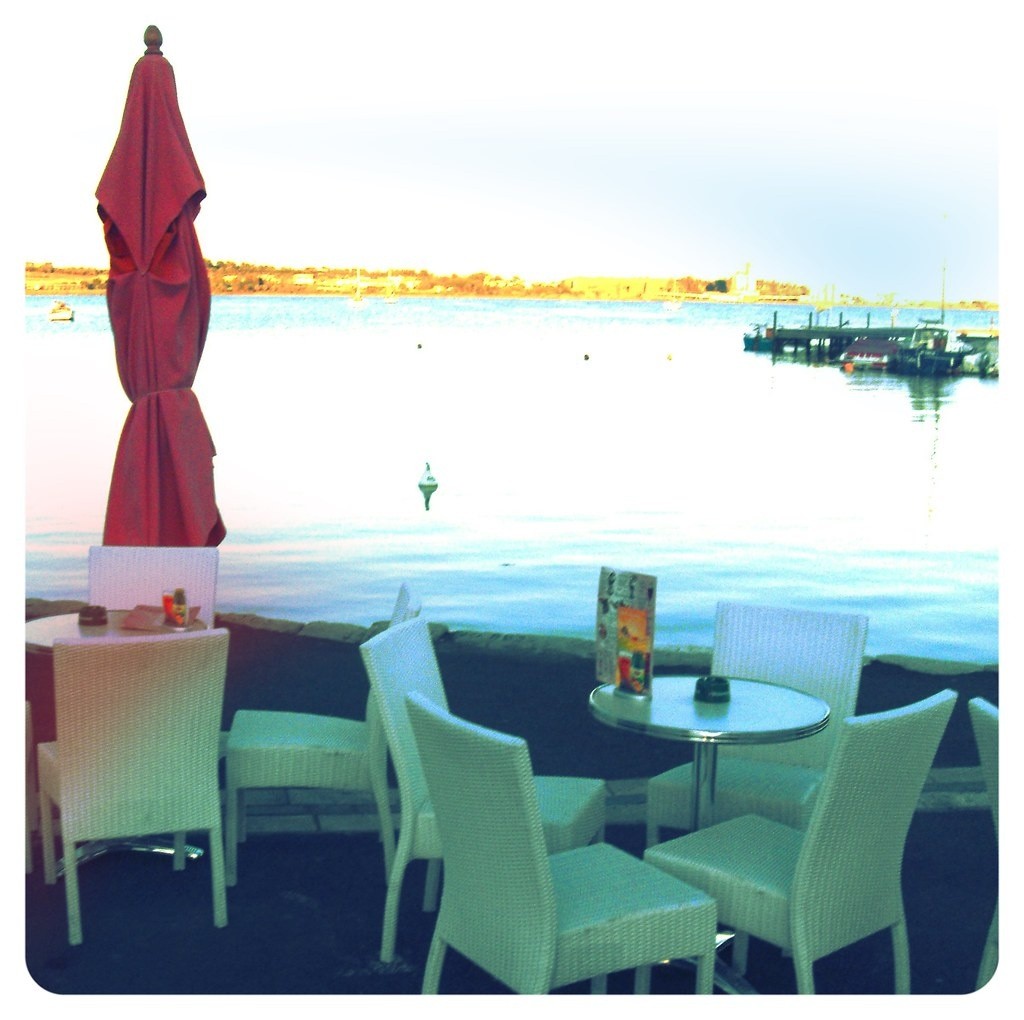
[403,688,718,995]
[225,579,424,887]
[37,627,230,945]
[86,545,220,629]
[645,595,872,961]
[968,696,999,989]
[355,614,606,964]
[640,688,962,994]
[26,701,39,875]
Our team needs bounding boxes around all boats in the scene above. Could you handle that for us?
[50,305,74,322]
[890,327,966,375]
[839,338,899,370]
[959,333,999,375]
[743,336,772,353]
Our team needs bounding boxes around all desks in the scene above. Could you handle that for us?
[26,609,211,878]
[587,673,830,995]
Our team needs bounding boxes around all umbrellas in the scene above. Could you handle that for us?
[96,26,226,548]
